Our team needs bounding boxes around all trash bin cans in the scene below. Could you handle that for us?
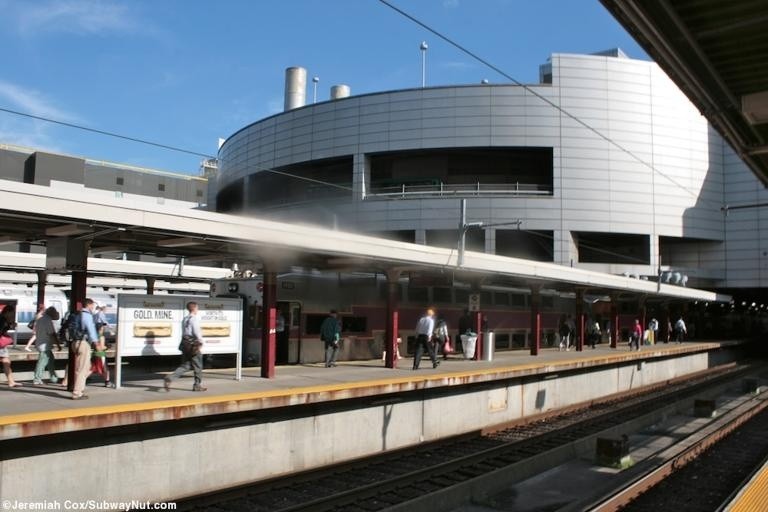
[461,334,478,359]
[242,330,263,367]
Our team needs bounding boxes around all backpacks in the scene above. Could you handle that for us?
[67,310,90,340]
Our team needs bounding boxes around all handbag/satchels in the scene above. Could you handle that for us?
[179,333,202,357]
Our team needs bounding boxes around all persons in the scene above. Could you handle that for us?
[434,314,449,362]
[413,309,440,369]
[382,326,402,362]
[558,313,596,351]
[276,306,288,364]
[320,309,342,368]
[481,313,488,331]
[628,315,688,352]
[459,309,473,350]
[0,305,23,388]
[24,297,114,399]
[164,302,208,391]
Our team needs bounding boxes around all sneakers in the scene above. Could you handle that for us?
[73,394,90,401]
[165,378,172,391]
[193,383,207,391]
[105,381,117,389]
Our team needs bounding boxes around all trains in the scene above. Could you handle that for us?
[209,270,767,365]
[0,280,117,343]
